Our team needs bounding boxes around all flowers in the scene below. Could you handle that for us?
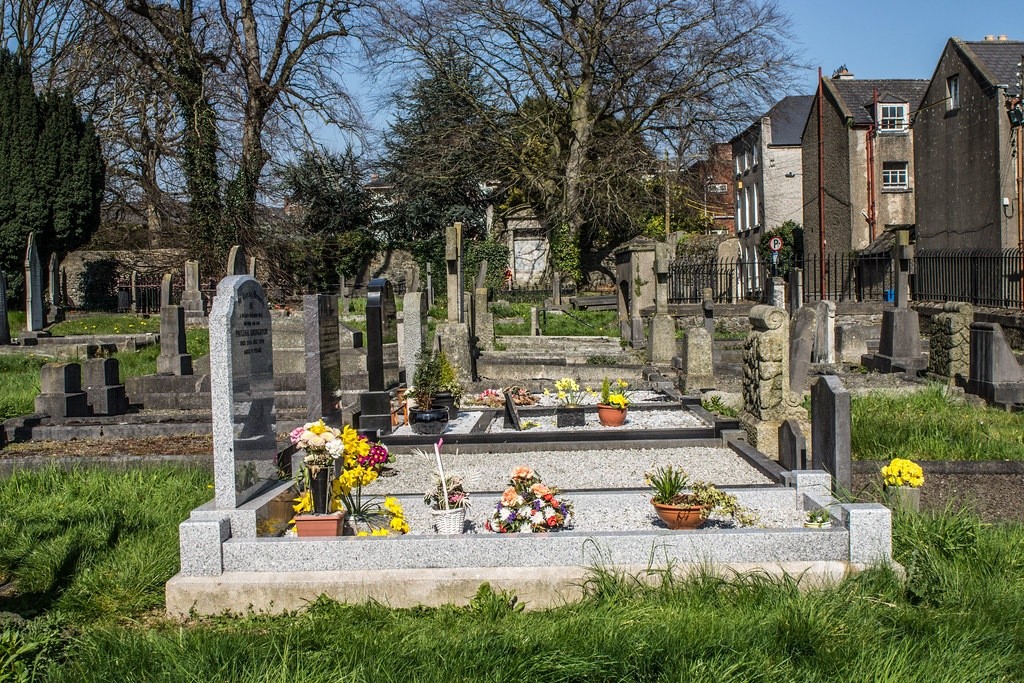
[600,376,631,410]
[423,471,472,514]
[543,376,599,406]
[485,463,577,533]
[643,460,767,528]
[880,458,925,488]
[288,418,410,537]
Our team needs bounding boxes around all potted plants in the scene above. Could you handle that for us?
[403,349,450,435]
[433,352,464,420]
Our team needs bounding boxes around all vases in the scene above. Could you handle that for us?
[430,508,467,534]
[649,494,714,531]
[885,484,921,517]
[342,511,375,536]
[596,402,628,427]
[307,465,332,515]
[294,511,346,537]
[556,405,586,428]
